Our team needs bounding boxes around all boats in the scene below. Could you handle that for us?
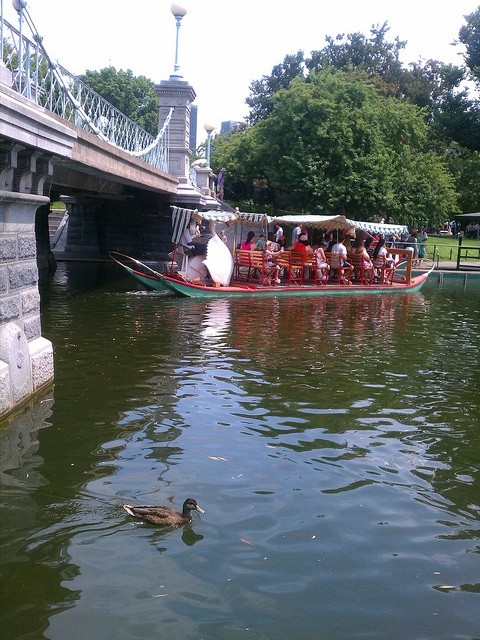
[103,208,439,298]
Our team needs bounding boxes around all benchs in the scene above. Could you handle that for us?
[292,251,328,282]
[346,254,372,285]
[325,252,353,285]
[237,249,277,285]
[374,256,394,284]
[272,251,303,286]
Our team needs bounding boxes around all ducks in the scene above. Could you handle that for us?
[123,498,204,526]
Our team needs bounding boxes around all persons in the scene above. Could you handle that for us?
[302,227,308,235]
[364,237,375,255]
[240,232,264,275]
[373,232,381,240]
[343,228,356,239]
[274,224,285,246]
[419,244,428,267]
[324,228,333,243]
[428,219,479,240]
[264,241,283,283]
[331,235,354,285]
[219,230,228,244]
[292,223,302,243]
[374,239,396,284]
[179,223,207,256]
[356,241,381,280]
[408,228,418,266]
[295,240,308,264]
[418,226,428,249]
[314,241,330,283]
[343,239,353,254]
[389,232,401,248]
[287,234,313,252]
[256,234,263,249]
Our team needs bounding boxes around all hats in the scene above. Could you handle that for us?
[299,235,307,240]
